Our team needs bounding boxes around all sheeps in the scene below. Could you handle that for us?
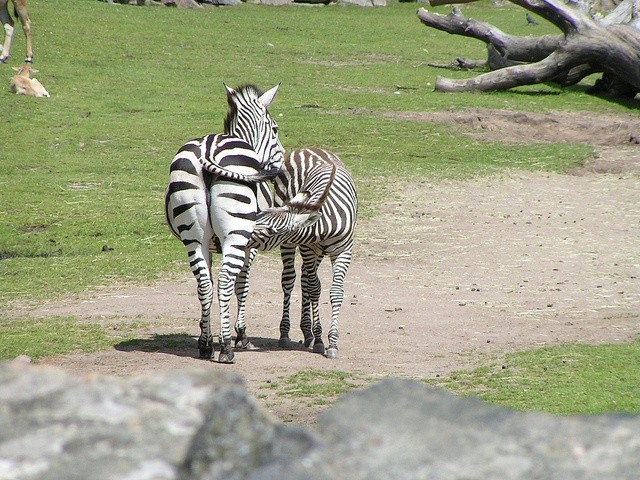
[10,65,51,98]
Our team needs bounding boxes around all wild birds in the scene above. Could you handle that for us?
[524,12,537,26]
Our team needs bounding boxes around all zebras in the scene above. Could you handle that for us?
[165,80,287,363]
[214,147,359,359]
